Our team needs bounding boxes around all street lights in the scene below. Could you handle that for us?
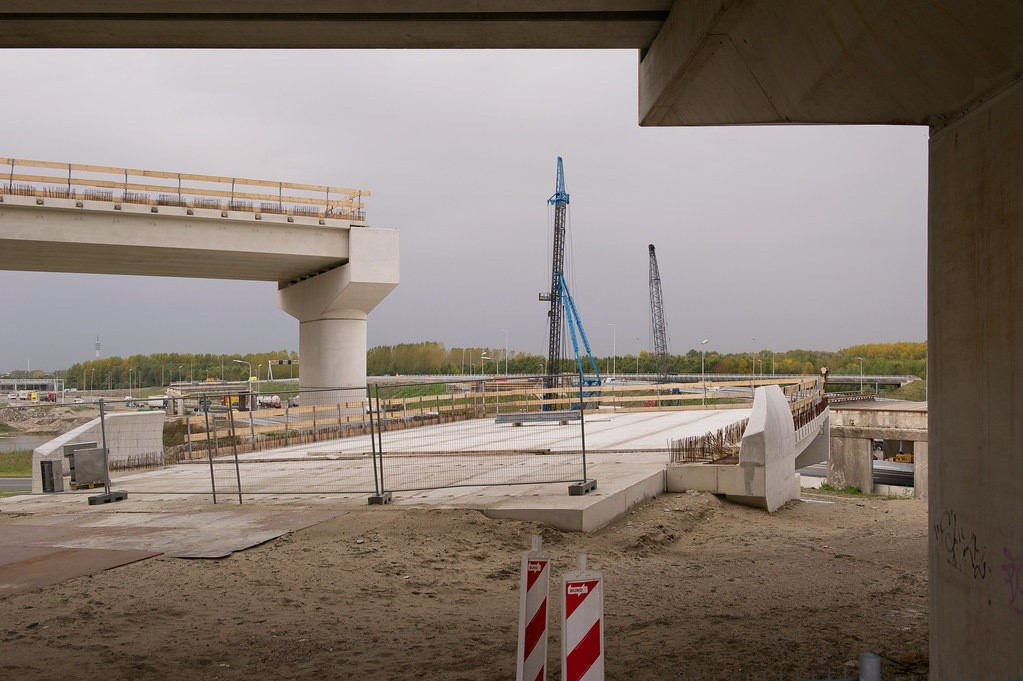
[481,357,498,414]
[481,351,487,411]
[608,324,616,378]
[758,359,762,386]
[91,359,251,429]
[499,329,508,376]
[858,357,862,394]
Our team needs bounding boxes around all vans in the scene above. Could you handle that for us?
[8,394,16,400]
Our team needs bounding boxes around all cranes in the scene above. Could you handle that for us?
[537,155,602,411]
[648,244,668,355]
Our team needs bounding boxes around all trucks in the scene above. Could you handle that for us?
[148,395,168,410]
